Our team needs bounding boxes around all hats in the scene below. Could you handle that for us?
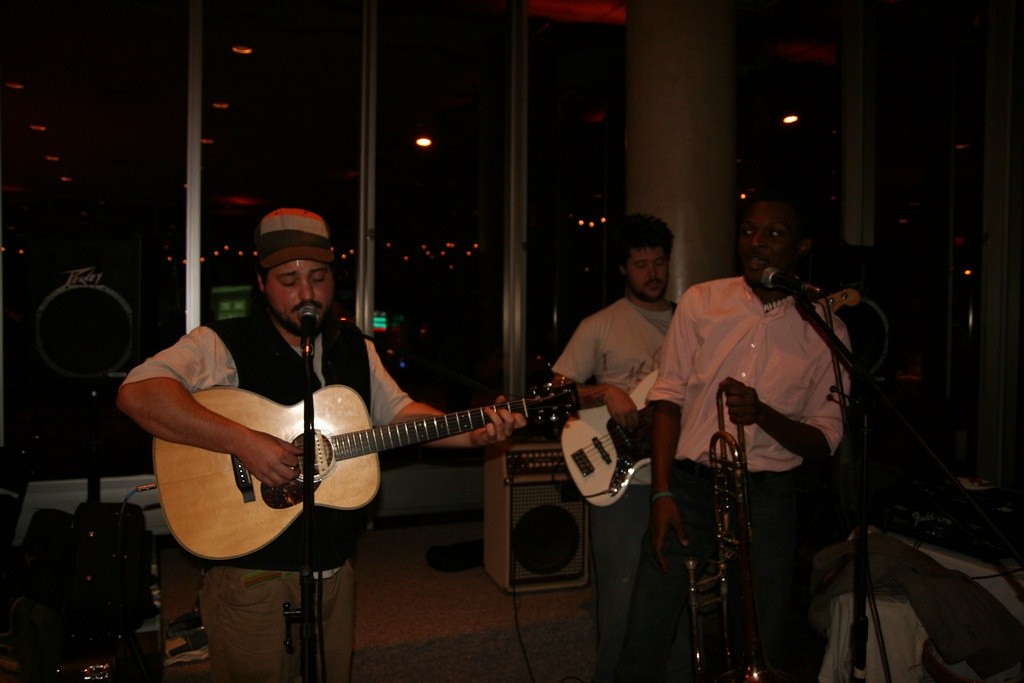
[254,208,335,269]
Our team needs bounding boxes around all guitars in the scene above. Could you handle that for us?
[152,377,580,562]
[560,286,863,508]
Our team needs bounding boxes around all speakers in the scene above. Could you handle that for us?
[481,438,591,590]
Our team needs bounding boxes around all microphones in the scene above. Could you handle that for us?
[298,304,321,359]
[761,266,822,294]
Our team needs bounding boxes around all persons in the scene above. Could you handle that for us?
[552,214,694,683]
[614,193,852,683]
[116,208,529,683]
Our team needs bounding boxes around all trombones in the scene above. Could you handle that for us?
[684,377,790,683]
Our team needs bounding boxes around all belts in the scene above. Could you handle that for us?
[673,459,793,481]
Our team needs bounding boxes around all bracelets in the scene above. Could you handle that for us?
[652,491,672,503]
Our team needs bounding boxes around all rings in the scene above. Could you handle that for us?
[634,412,639,416]
